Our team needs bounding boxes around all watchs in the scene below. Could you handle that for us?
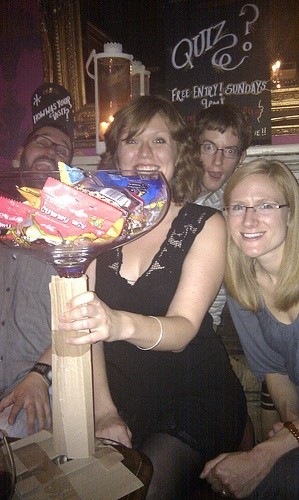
[29,362,52,386]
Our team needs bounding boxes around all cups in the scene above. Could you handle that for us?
[0,429,16,500]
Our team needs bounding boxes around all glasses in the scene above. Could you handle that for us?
[24,135,73,158]
[223,203,287,216]
[199,144,242,159]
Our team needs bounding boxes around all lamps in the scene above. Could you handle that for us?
[86,39,133,154]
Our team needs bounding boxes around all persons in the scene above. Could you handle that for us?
[0,118,75,438]
[58,95,259,500]
[188,104,253,334]
[196,159,299,500]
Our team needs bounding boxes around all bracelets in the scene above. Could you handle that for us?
[283,421,299,442]
[137,316,163,351]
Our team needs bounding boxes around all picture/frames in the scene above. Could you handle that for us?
[38,0,299,147]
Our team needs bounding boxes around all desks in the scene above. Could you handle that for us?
[0,437,154,500]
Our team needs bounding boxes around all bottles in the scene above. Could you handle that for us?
[131,59,151,101]
[85,41,133,153]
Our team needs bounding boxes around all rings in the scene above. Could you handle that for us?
[220,489,227,497]
